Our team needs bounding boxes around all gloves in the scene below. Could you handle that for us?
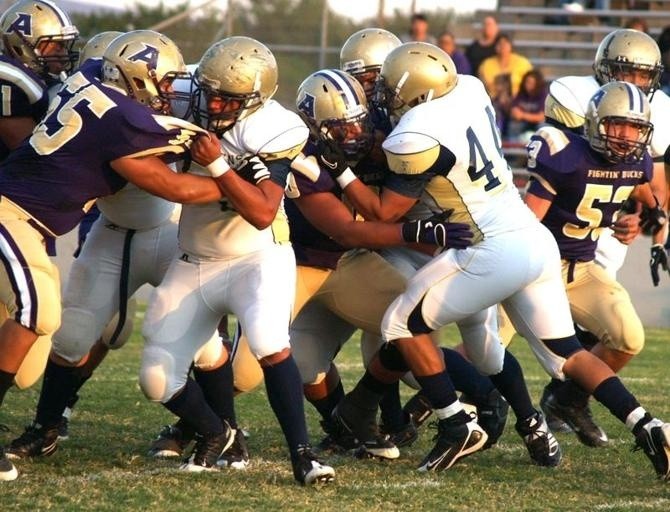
[229,152,271,185]
[315,138,347,179]
[638,195,668,286]
[402,209,474,249]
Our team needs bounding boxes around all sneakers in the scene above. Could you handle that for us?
[476,389,509,452]
[629,418,670,483]
[514,410,562,467]
[416,408,488,475]
[355,423,402,461]
[540,400,574,434]
[293,443,336,487]
[332,394,376,462]
[540,384,608,448]
[146,418,251,472]
[378,410,418,448]
[1,406,71,481]
[312,435,339,462]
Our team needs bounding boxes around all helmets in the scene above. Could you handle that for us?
[0,0,278,139]
[295,28,457,158]
[584,28,664,166]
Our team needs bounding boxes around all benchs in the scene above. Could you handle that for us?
[449,1,669,82]
